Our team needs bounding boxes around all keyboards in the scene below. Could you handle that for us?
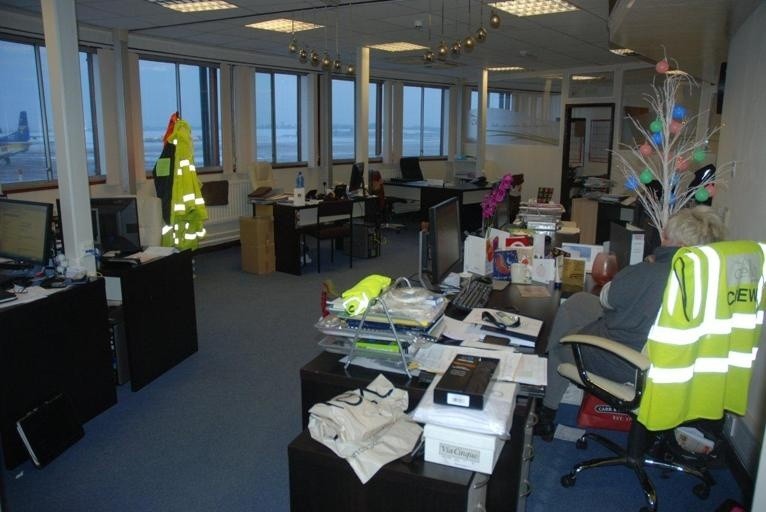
[450,280,492,311]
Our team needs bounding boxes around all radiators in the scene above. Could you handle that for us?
[200,178,255,227]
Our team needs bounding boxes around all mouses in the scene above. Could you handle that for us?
[477,276,493,283]
[40,277,66,288]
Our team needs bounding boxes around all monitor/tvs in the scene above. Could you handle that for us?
[349,162,367,198]
[491,181,510,229]
[0,198,54,270]
[453,160,479,180]
[419,196,462,292]
[56,196,143,267]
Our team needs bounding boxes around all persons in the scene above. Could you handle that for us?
[533,204,725,442]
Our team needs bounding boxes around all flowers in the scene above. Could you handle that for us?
[477,169,516,237]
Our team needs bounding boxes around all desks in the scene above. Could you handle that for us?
[252,190,382,277]
[569,196,635,244]
[379,174,495,225]
[298,260,561,512]
[0,262,117,477]
[96,245,200,392]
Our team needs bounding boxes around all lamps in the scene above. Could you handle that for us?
[283,1,503,77]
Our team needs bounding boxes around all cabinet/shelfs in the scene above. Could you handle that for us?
[280,420,492,512]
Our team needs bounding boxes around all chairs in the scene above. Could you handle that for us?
[347,195,386,258]
[366,167,408,233]
[550,238,766,510]
[107,298,132,387]
[305,201,355,273]
[400,157,425,179]
[247,161,278,192]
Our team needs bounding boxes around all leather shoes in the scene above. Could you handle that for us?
[533,422,554,442]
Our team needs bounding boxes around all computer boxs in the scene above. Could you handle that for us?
[108,305,130,385]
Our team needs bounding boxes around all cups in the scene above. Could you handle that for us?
[72,270,85,281]
[357,188,363,196]
[44,266,55,276]
[510,262,533,283]
[590,251,619,286]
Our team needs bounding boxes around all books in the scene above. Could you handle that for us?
[519,202,566,231]
[315,288,449,343]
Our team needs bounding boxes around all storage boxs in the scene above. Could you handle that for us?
[236,214,276,275]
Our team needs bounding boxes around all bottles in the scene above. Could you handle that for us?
[296,171,304,188]
[83,247,97,277]
